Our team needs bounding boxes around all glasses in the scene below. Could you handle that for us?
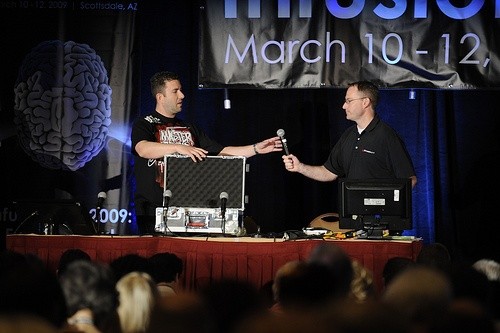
[345,96,372,103]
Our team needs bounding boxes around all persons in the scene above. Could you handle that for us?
[281,80,418,190]
[132,72,284,233]
[0,239,500,333]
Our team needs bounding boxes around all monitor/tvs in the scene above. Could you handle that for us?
[336,178,414,240]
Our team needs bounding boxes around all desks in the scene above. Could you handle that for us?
[5,234,423,296]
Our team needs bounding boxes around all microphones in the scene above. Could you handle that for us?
[276,128,290,160]
[14,210,39,234]
[164,189,173,218]
[96,191,106,219]
[220,191,228,218]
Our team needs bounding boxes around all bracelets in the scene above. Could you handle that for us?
[253,144,258,154]
